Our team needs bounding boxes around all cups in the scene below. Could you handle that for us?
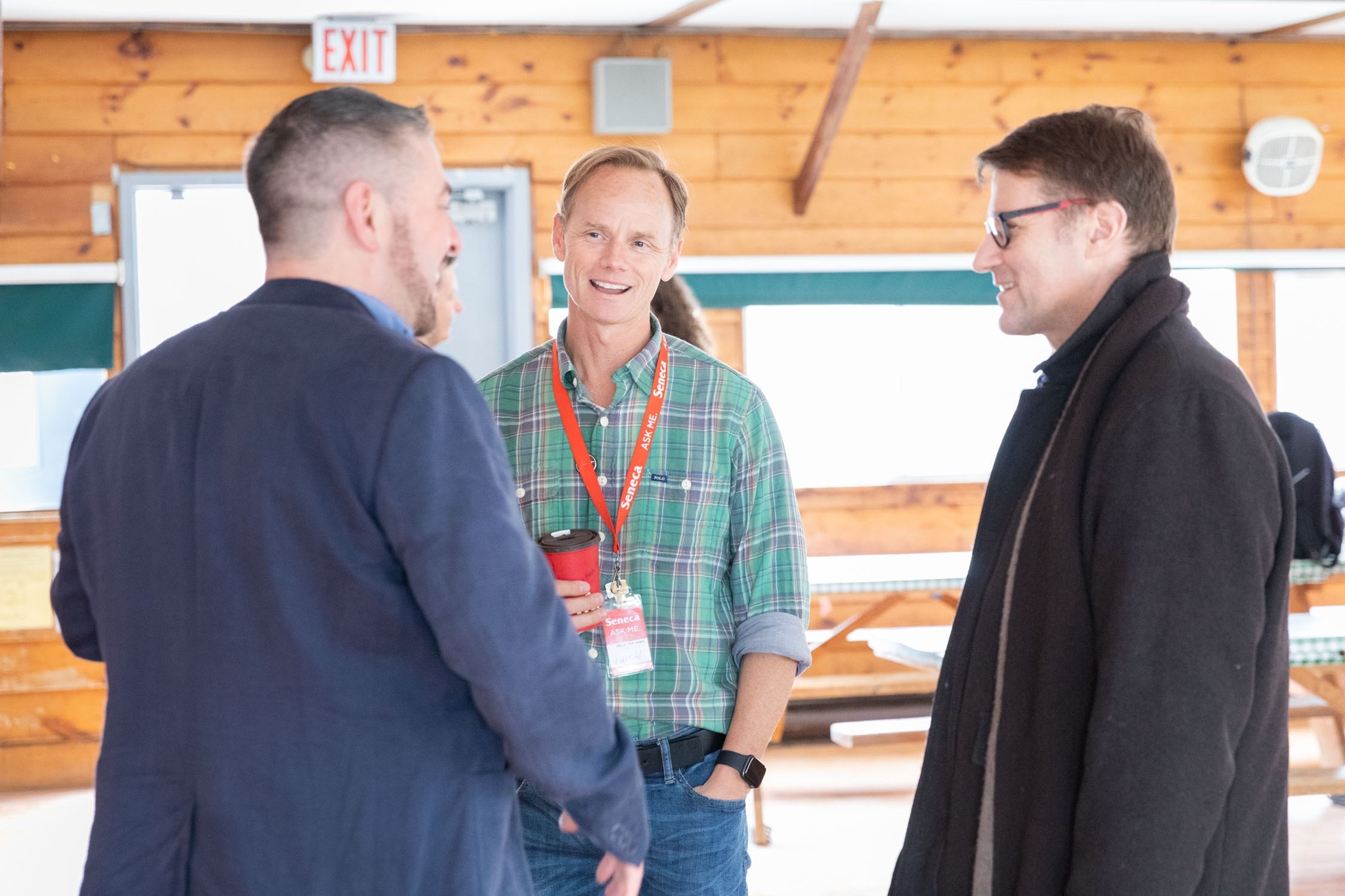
[536,528,602,631]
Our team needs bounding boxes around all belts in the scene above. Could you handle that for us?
[632,728,715,774]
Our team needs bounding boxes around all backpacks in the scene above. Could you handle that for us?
[1267,412,1345,567]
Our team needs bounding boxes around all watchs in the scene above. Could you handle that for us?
[716,750,767,788]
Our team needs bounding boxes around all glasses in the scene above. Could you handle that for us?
[984,198,1094,248]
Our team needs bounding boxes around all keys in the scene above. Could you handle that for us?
[605,580,630,601]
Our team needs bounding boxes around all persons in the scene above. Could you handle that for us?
[651,275,713,355]
[889,102,1296,896]
[50,88,648,896]
[472,144,811,896]
[416,257,462,348]
